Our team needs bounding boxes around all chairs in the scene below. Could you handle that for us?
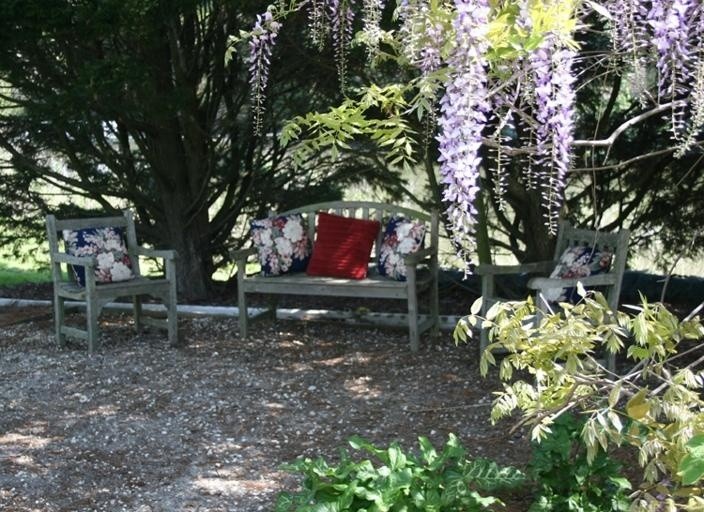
[475,221,633,382]
[44,212,179,354]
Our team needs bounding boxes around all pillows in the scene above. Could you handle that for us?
[546,245,612,302]
[249,213,312,278]
[61,226,134,285]
[306,212,382,280]
[379,217,426,280]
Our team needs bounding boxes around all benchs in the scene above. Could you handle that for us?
[232,200,440,353]
